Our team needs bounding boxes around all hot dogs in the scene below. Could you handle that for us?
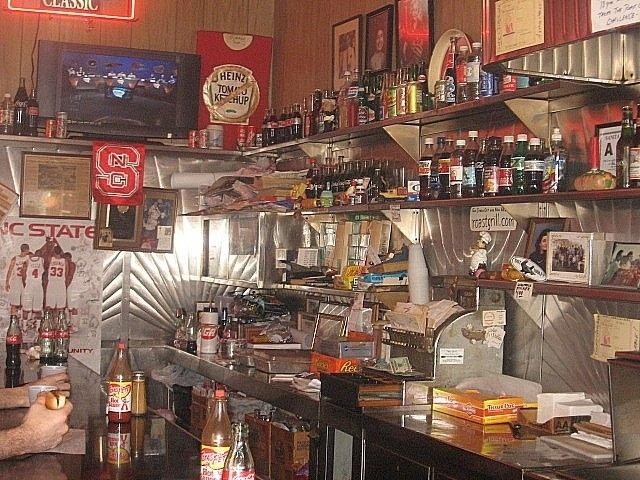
[37,389,65,409]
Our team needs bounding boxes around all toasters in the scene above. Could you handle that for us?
[130,373,148,417]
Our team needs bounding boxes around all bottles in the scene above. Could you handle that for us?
[199,388,232,480]
[174,302,246,367]
[0,78,39,137]
[223,422,255,480]
[616,104,639,190]
[130,418,145,459]
[6,315,22,371]
[5,369,21,389]
[266,36,525,147]
[105,424,131,479]
[39,309,70,363]
[291,128,563,203]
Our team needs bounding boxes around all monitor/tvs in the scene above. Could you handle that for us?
[36,39,201,146]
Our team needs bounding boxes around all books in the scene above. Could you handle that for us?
[538,421,614,460]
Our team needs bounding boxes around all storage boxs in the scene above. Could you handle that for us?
[190,390,207,431]
[245,412,272,480]
[271,420,311,480]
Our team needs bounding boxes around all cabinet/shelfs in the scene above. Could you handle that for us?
[362,441,460,480]
[200,80,640,369]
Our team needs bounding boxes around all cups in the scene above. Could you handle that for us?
[40,366,65,378]
[405,243,430,308]
[28,385,55,406]
[106,342,132,424]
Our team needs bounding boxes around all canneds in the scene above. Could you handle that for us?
[198,129,210,148]
[435,80,446,108]
[189,130,198,149]
[45,119,56,138]
[387,80,420,117]
[56,112,68,138]
[236,125,262,152]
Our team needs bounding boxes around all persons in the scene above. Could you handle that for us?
[401,0,423,63]
[552,244,589,270]
[605,250,640,286]
[68,65,177,86]
[369,27,385,72]
[6,237,84,334]
[529,226,552,268]
[0,372,71,459]
[143,198,172,240]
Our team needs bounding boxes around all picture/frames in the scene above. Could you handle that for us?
[524,217,567,271]
[364,4,394,77]
[101,191,142,248]
[595,119,636,178]
[20,150,92,219]
[295,295,380,359]
[93,187,177,253]
[546,231,592,285]
[394,0,435,69]
[332,14,362,95]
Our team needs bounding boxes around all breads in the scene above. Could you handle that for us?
[574,168,617,191]
